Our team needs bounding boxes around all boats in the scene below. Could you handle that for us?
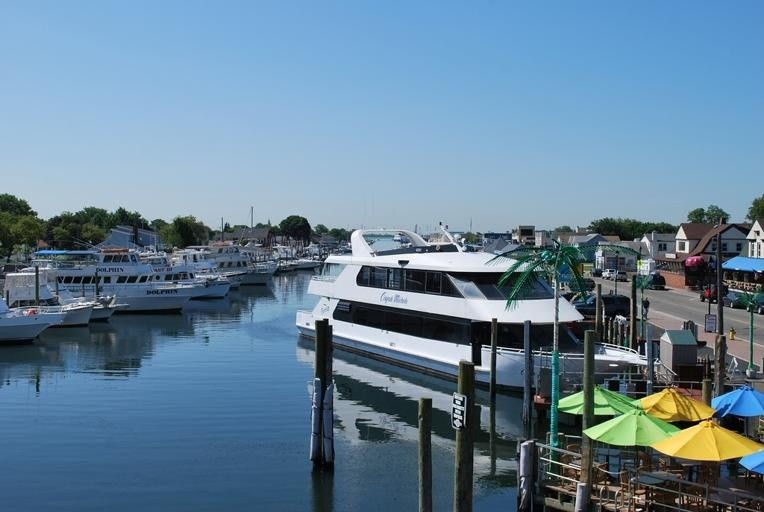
[189,266,320,318]
[0,207,324,342]
[294,336,521,493]
[0,310,198,374]
[295,226,661,394]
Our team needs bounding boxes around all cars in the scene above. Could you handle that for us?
[590,268,601,276]
[722,292,745,309]
[748,295,764,315]
[633,275,647,286]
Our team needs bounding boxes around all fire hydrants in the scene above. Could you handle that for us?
[729,328,736,340]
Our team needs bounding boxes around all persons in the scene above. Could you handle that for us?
[643,298,650,319]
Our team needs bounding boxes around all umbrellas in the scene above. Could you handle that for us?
[557,382,764,490]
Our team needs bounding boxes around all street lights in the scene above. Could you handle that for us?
[743,289,759,379]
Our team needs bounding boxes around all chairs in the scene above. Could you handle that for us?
[561,444,764,512]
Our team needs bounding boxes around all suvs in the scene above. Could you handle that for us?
[642,272,665,290]
[601,268,616,279]
[610,271,628,282]
[700,283,728,304]
[571,293,631,319]
